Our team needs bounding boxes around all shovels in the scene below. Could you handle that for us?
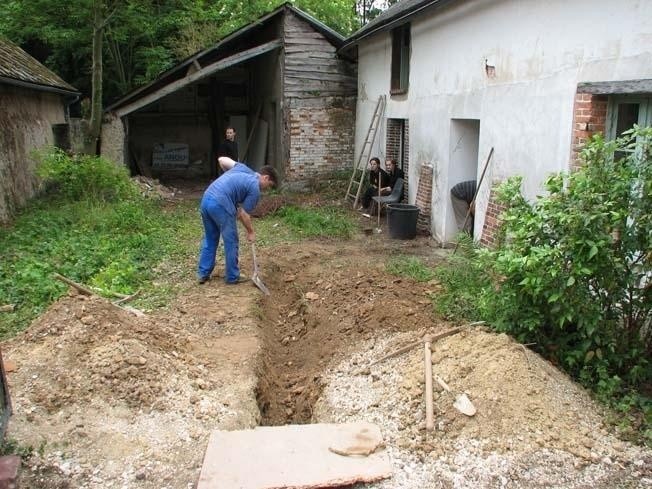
[252,243,271,297]
[432,374,477,417]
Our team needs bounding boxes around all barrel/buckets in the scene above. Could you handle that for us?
[385,203,420,239]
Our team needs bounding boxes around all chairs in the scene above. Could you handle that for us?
[371,178,405,227]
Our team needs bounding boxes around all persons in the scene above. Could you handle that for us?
[218,127,239,163]
[198,155,279,285]
[364,159,404,219]
[449,179,476,237]
[356,157,388,210]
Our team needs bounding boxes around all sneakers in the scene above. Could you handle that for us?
[357,206,371,218]
[226,273,249,283]
[198,271,213,283]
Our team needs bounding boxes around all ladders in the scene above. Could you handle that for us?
[345,94,386,209]
